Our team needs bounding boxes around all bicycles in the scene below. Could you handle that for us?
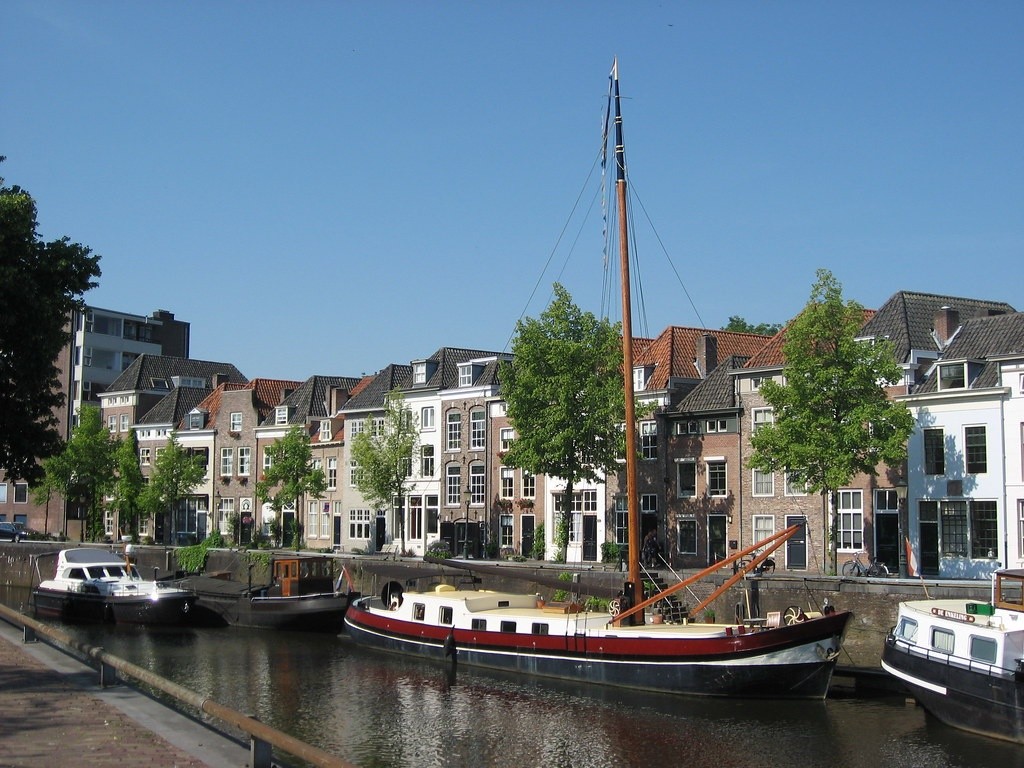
[841,550,889,578]
[736,551,776,576]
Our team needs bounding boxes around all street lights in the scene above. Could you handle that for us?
[215,491,222,530]
[463,483,472,561]
[79,494,86,542]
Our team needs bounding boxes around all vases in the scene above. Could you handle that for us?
[502,508,508,513]
[237,481,241,485]
[537,600,546,608]
[523,508,528,514]
[654,614,663,624]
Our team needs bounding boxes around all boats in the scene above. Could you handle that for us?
[28,546,199,629]
[881,567,1024,745]
[167,552,350,634]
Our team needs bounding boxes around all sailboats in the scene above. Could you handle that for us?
[340,55,856,702]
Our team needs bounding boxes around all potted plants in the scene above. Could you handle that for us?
[258,543,271,549]
[57,531,66,542]
[584,596,610,613]
[601,541,622,573]
[704,610,716,624]
[529,520,545,560]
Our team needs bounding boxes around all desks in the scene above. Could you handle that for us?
[743,618,767,632]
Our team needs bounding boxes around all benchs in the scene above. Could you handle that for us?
[373,544,398,561]
[744,625,774,631]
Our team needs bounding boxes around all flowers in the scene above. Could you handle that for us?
[517,498,534,508]
[496,499,512,508]
[236,477,244,481]
[227,431,239,438]
[242,517,254,524]
[217,476,230,483]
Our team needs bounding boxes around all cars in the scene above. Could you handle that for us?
[0,521,36,543]
